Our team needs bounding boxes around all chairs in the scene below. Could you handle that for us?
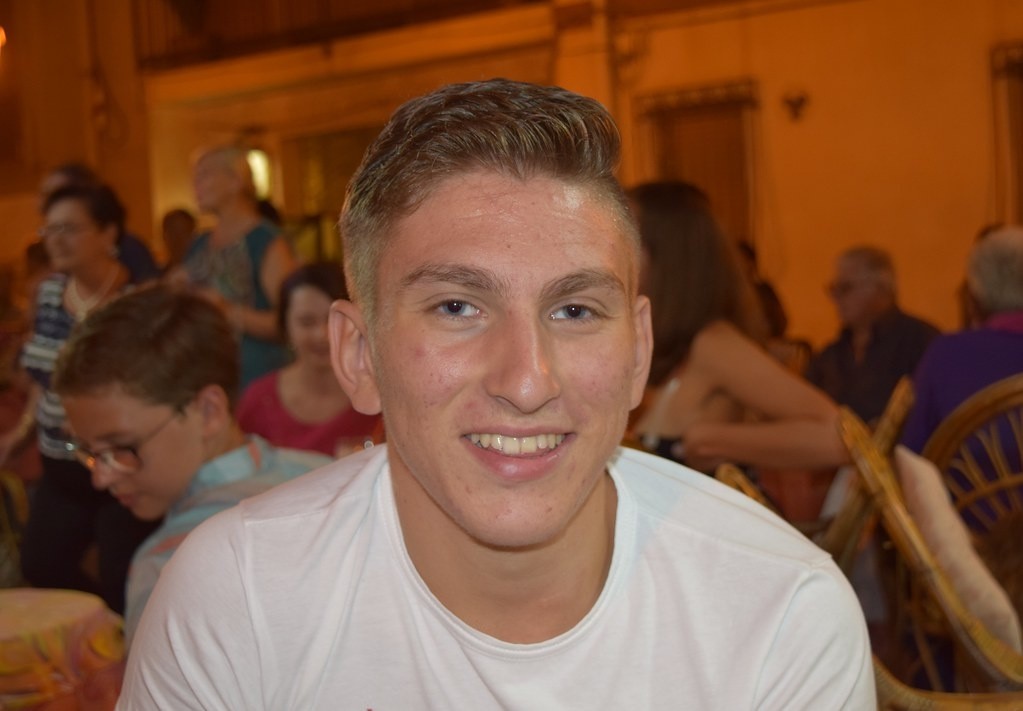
[717,371,1023,710]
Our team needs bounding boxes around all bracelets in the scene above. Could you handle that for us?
[15,411,39,438]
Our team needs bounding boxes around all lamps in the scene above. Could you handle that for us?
[235,120,284,227]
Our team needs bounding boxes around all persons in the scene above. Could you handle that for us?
[622,177,873,504]
[731,220,1023,529]
[56,283,344,662]
[21,159,163,284]
[0,183,158,622]
[114,79,878,710]
[158,138,300,408]
[243,265,391,466]
[1,273,57,542]
[157,205,205,277]
[894,221,1023,694]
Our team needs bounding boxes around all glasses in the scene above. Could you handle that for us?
[62,402,187,474]
